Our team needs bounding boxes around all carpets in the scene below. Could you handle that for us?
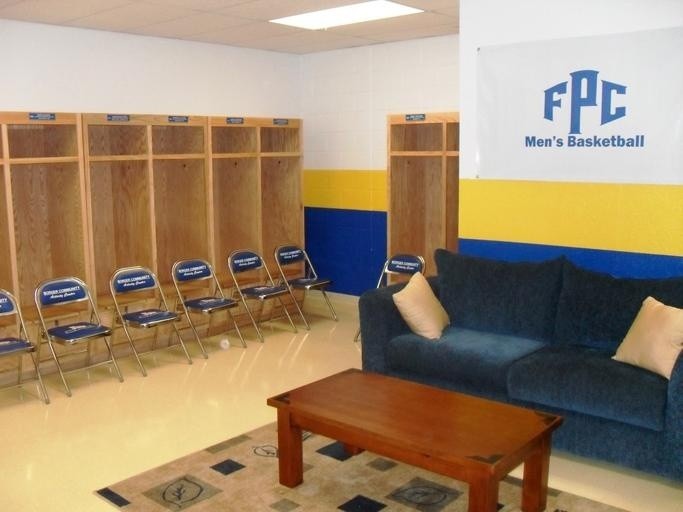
[94,419,630,512]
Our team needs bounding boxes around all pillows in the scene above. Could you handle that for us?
[392,271,452,341]
[609,296,683,380]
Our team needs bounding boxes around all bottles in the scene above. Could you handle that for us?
[220,335,229,350]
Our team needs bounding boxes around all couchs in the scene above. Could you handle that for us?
[359,248,683,483]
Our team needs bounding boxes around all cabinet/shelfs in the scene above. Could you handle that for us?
[386,111,460,286]
[0,117,303,388]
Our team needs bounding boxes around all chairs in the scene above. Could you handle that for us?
[222,247,311,343]
[353,253,428,345]
[268,243,339,330]
[105,266,193,376]
[166,257,247,360]
[31,276,124,397]
[0,289,50,405]
